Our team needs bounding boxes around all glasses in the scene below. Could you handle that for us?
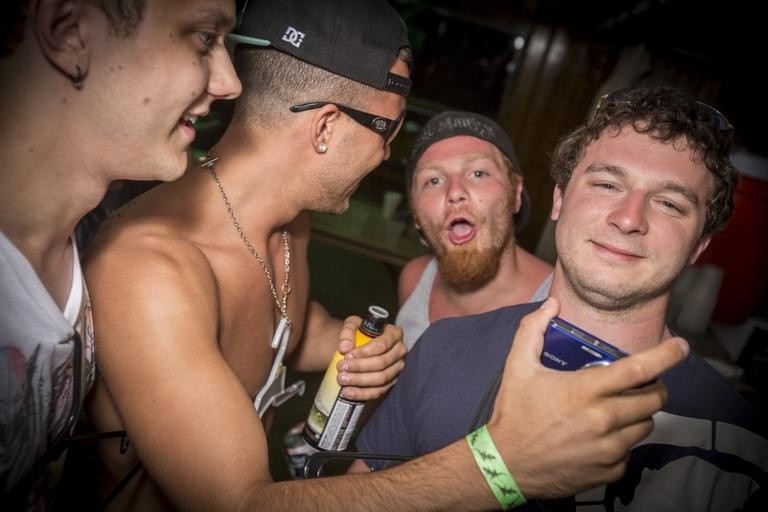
[596,88,734,143]
[288,99,407,149]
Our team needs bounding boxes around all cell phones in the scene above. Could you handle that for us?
[539,316,631,371]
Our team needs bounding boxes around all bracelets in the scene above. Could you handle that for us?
[464,424,527,510]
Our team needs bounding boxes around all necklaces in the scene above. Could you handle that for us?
[199,157,292,318]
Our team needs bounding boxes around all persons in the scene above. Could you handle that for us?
[0,0,244,512]
[344,87,768,512]
[393,109,556,359]
[80,0,691,511]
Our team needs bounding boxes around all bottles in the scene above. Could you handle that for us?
[301,305,389,454]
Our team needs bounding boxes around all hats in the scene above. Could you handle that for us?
[404,110,530,246]
[226,2,416,100]
